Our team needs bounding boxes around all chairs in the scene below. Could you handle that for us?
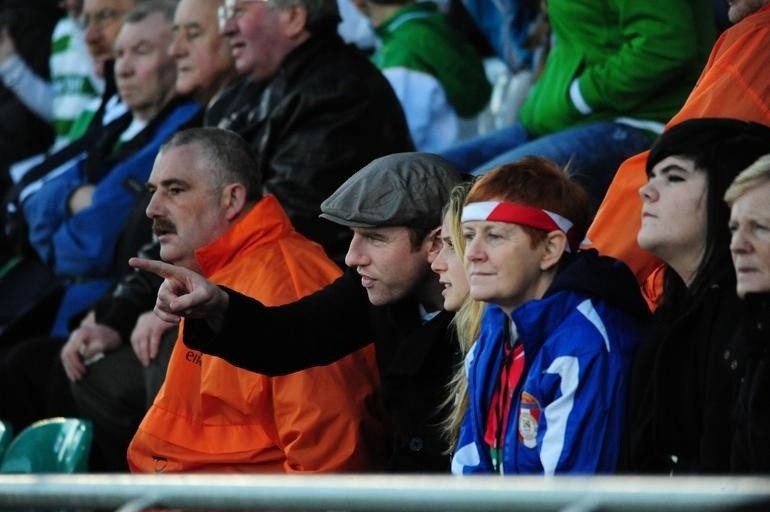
[1,417,92,472]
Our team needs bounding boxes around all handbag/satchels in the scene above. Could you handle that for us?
[0,231,63,351]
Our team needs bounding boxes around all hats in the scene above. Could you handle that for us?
[318,153,464,230]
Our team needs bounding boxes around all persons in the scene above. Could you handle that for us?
[1,1,769,478]
